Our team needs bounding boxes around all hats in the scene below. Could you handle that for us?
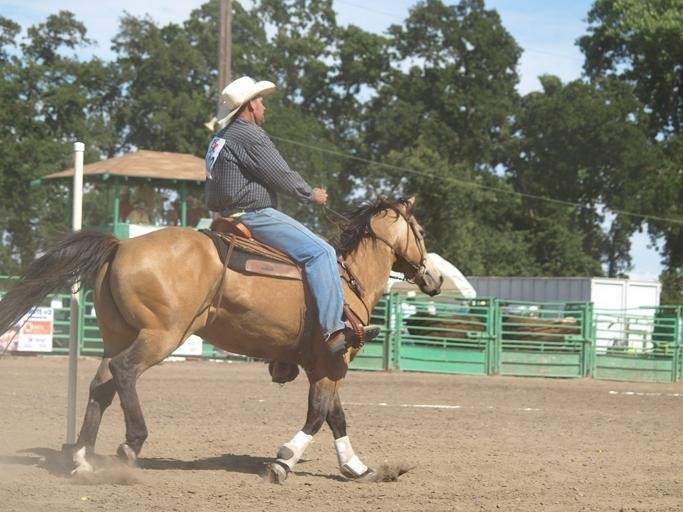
[203,76,276,133]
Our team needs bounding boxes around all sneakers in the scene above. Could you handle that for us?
[273,359,291,378]
[327,325,380,354]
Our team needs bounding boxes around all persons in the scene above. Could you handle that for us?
[205,74,380,356]
[127,199,150,226]
[177,195,206,226]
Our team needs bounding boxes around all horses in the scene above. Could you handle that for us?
[0,189,445,486]
